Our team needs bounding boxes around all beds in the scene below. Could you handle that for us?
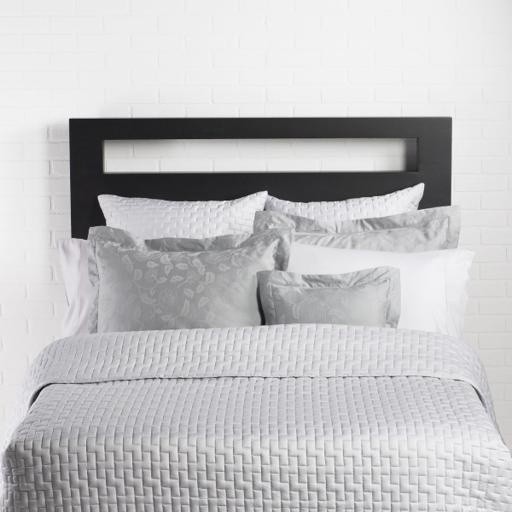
[1,116,512,507]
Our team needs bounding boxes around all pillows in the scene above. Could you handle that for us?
[264,183,427,224]
[256,263,403,328]
[94,188,269,250]
[280,241,483,331]
[252,203,467,250]
[81,223,292,331]
